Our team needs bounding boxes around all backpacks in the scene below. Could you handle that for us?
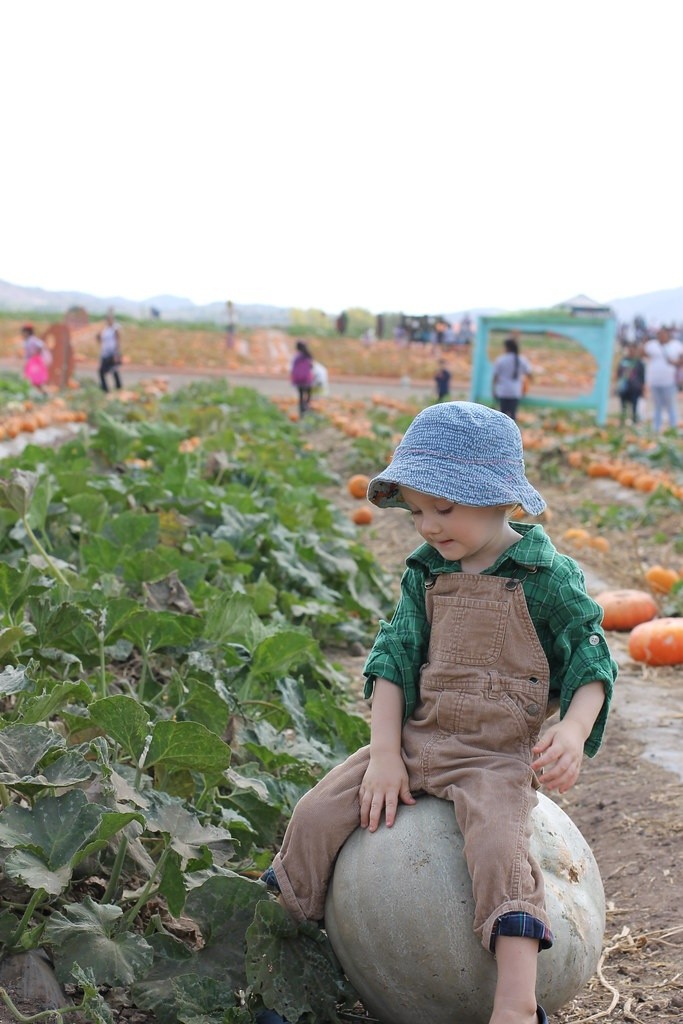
[293,357,311,384]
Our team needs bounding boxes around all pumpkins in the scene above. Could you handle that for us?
[324,780,606,1024]
[0,316,683,667]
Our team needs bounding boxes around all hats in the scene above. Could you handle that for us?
[367,400,548,517]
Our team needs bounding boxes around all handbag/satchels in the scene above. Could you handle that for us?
[615,378,629,391]
[309,360,329,396]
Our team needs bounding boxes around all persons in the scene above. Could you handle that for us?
[97,317,124,391]
[338,312,347,335]
[376,314,474,345]
[266,399,618,1024]
[224,301,239,333]
[492,339,534,421]
[616,314,683,428]
[435,359,451,402]
[291,341,315,415]
[22,324,53,397]
